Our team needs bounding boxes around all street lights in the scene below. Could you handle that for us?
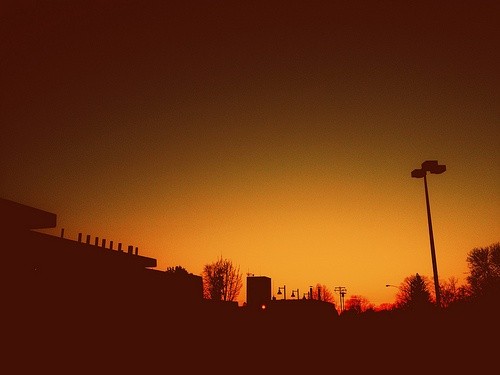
[408,159,448,308]
[386,284,411,297]
[266,284,350,318]
